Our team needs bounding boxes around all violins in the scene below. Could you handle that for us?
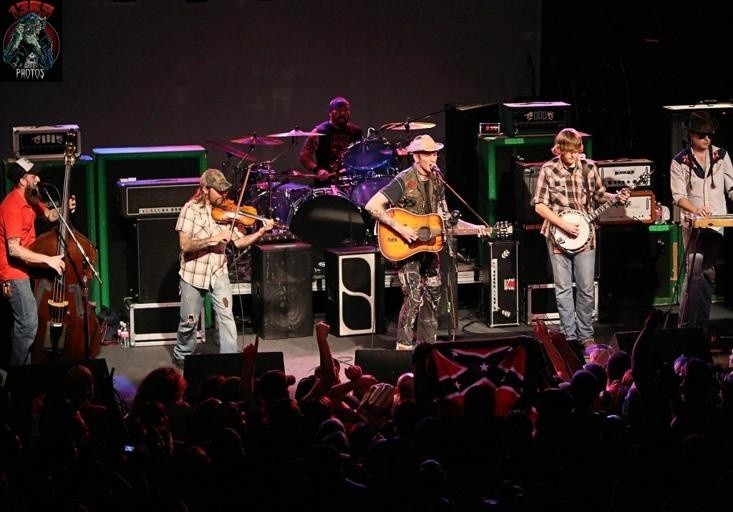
[212,198,291,231]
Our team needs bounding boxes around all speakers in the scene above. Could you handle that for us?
[251,243,314,340]
[353,350,413,400]
[607,327,714,364]
[5,359,130,443]
[444,102,499,258]
[324,246,385,337]
[184,352,285,407]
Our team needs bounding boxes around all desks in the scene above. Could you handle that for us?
[479,135,592,266]
[96,144,208,313]
[0,156,100,316]
[599,224,725,308]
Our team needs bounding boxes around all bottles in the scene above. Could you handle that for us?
[117,320,130,348]
[654,202,662,224]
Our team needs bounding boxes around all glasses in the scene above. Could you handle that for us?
[696,132,713,139]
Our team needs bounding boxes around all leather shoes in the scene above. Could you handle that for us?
[172,356,184,370]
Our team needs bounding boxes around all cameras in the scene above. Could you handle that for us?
[124,445,135,452]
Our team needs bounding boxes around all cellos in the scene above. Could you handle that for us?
[26,140,103,367]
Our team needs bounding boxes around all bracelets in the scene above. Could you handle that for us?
[312,166,322,176]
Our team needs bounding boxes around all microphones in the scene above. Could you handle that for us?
[33,182,49,187]
[430,164,440,172]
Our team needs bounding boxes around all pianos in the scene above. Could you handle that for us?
[683,214,733,230]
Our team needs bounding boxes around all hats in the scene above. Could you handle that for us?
[405,134,444,153]
[6,157,43,184]
[681,110,719,134]
[199,168,232,192]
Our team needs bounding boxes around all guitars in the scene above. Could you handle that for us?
[377,208,512,262]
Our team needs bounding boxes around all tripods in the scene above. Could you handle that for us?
[256,175,289,234]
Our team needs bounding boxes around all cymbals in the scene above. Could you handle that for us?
[202,139,256,162]
[264,129,328,139]
[284,168,319,181]
[229,136,284,147]
[381,121,436,132]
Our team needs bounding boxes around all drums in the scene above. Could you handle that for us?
[348,173,395,206]
[270,183,363,249]
[341,138,397,172]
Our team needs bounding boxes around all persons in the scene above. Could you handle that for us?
[0,317,732,511]
[174,168,273,379]
[299,97,365,187]
[534,128,630,357]
[0,158,77,366]
[364,135,493,351]
[669,113,733,330]
